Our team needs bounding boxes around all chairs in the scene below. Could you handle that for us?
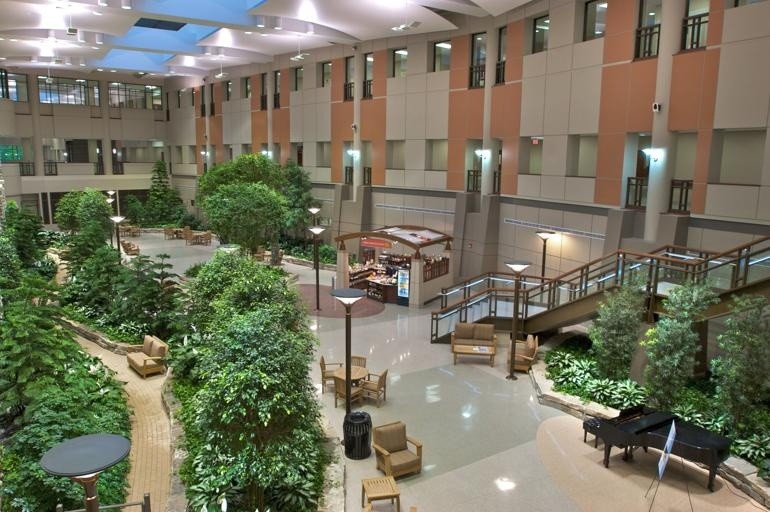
[320,355,344,394]
[372,421,422,479]
[333,376,361,408]
[359,368,388,408]
[120,224,282,267]
[352,356,366,368]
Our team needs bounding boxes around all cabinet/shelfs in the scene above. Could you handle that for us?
[349,253,411,303]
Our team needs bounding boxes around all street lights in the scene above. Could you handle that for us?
[330,289,367,412]
[307,206,327,310]
[504,260,531,377]
[536,231,556,303]
[39,434,150,512]
[106,191,126,266]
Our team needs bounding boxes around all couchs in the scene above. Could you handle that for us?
[508,334,539,373]
[127,334,169,379]
[451,323,497,353]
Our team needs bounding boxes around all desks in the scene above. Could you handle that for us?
[453,345,495,368]
[334,366,368,388]
[362,475,400,512]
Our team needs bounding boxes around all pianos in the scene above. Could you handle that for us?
[600,405,732,491]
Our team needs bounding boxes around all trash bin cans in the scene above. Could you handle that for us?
[344,412,372,460]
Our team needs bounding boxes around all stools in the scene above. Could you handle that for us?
[583,416,608,449]
